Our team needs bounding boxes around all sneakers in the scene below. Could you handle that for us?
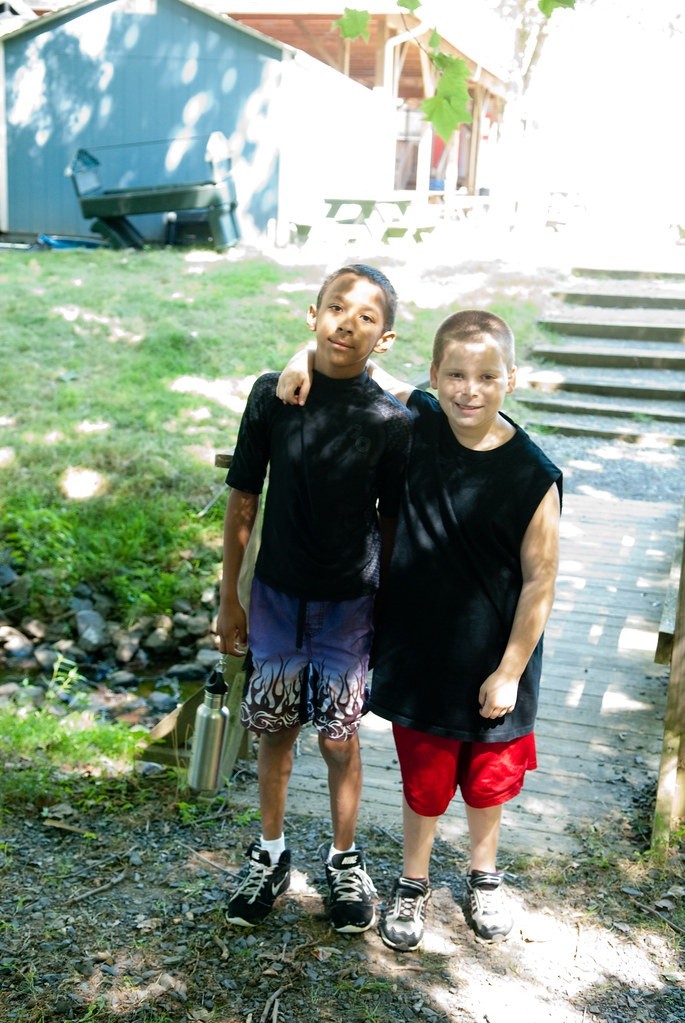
[226,843,292,927]
[465,864,514,945]
[319,841,380,933]
[379,873,431,951]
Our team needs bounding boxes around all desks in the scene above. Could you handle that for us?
[322,191,446,251]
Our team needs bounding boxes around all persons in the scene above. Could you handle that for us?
[216,264,414,931]
[275,310,563,952]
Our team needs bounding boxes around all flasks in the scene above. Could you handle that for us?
[186,669,229,790]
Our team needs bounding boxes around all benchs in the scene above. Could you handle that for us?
[290,219,440,237]
